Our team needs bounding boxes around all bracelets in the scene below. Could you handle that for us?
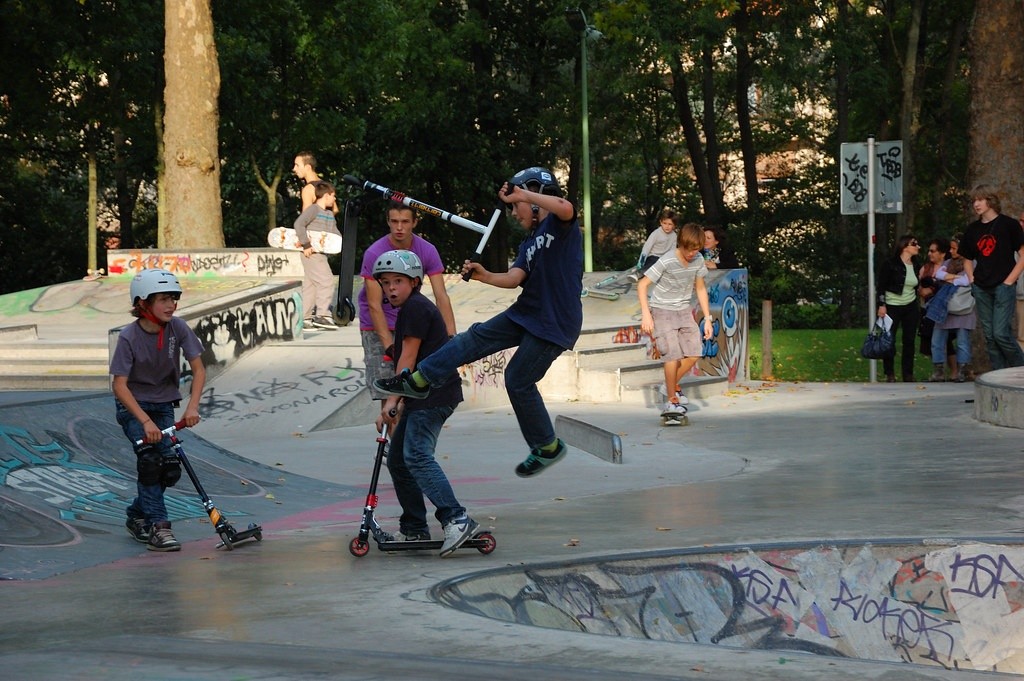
[449,333,457,339]
[142,419,151,426]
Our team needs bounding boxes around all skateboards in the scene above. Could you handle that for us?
[334,198,363,327]
[660,406,689,427]
[266,225,342,255]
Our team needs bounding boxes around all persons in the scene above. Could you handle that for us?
[627,212,678,282]
[698,226,739,269]
[373,166,583,479]
[293,150,339,217]
[636,221,714,415]
[371,249,480,558]
[293,180,339,333]
[877,219,1024,383]
[962,184,1024,372]
[108,269,206,552]
[358,200,458,466]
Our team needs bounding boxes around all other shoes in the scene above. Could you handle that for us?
[627,273,638,283]
[922,368,945,381]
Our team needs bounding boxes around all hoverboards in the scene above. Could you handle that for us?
[132,415,264,550]
[580,253,646,301]
[331,171,514,328]
[347,406,498,557]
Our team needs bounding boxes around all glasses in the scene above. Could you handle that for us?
[908,242,919,247]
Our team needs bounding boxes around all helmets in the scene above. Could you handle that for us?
[509,167,558,186]
[373,249,423,285]
[130,268,182,307]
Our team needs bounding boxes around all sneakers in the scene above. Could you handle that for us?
[302,318,325,331]
[659,384,689,405]
[515,439,567,479]
[374,368,430,399]
[312,316,338,329]
[662,403,687,413]
[393,525,431,541]
[124,507,150,543]
[439,511,481,558]
[146,521,180,552]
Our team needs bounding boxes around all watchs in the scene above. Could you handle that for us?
[704,315,712,322]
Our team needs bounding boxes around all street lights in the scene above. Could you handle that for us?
[564,10,593,273]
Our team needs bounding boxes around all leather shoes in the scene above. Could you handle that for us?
[888,374,896,382]
[904,376,916,382]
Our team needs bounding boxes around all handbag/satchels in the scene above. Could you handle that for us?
[862,318,896,359]
